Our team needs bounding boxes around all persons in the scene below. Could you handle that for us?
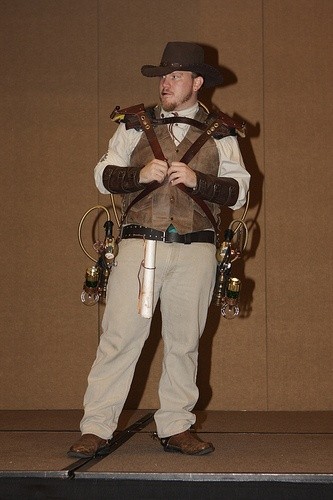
[66,41,250,460]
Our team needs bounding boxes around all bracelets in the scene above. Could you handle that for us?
[192,188,197,191]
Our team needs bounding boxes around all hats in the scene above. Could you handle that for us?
[141,41,226,89]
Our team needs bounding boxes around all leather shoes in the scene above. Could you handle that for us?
[164,430,213,457]
[68,434,108,458]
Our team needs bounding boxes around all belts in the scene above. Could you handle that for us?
[122,224,215,245]
